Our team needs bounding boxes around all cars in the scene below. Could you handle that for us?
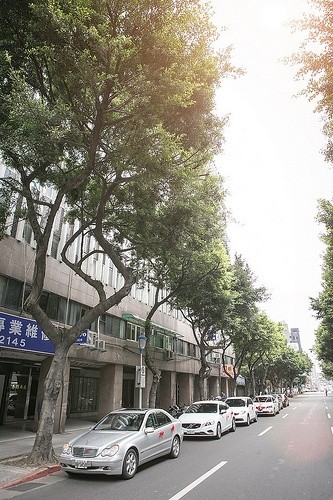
[58,408,185,480]
[223,396,258,426]
[252,393,290,416]
[178,400,236,441]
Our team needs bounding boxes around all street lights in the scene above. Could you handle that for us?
[137,333,148,409]
